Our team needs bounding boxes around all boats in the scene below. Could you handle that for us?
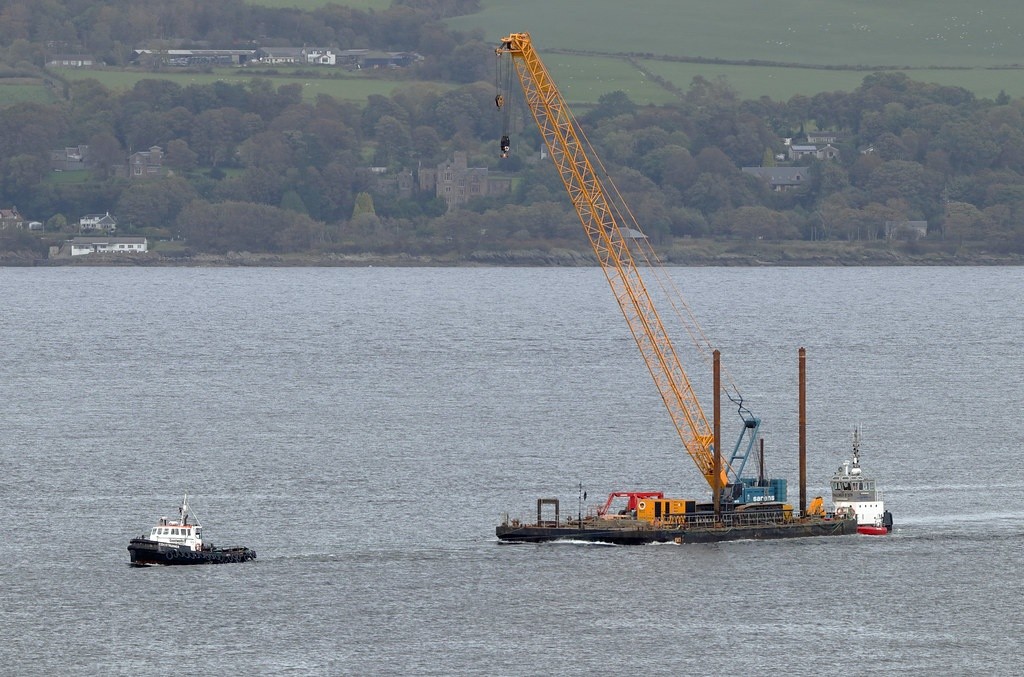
[828,426,894,536]
[492,342,859,545]
[127,493,257,569]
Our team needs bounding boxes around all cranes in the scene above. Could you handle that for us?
[492,32,795,526]
